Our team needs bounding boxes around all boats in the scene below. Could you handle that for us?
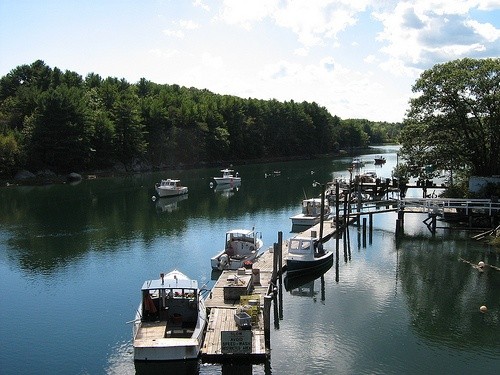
[284,234,333,280]
[288,198,331,226]
[154,179,188,198]
[324,170,378,203]
[374,158,386,164]
[132,269,208,361]
[208,168,241,190]
[210,228,265,271]
[352,158,363,167]
